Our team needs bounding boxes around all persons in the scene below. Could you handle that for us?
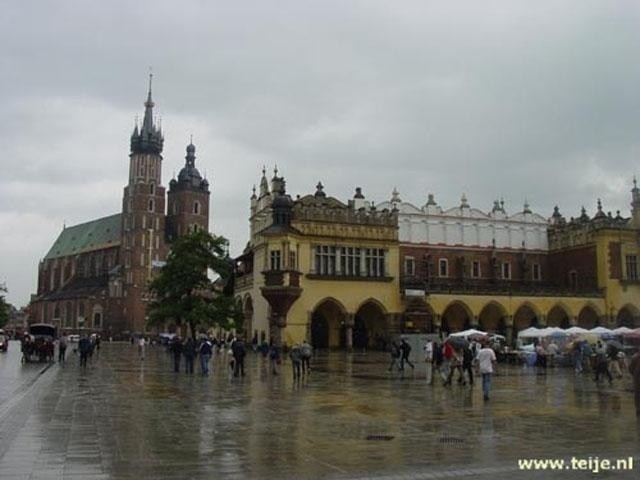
[137,335,145,361]
[58,330,68,361]
[77,333,92,370]
[628,351,640,418]
[172,333,313,381]
[387,333,628,402]
[95,334,101,350]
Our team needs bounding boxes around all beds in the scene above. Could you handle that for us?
[450,329,505,338]
[518,326,640,340]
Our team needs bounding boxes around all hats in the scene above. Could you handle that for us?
[0,323,101,363]
[109,329,179,346]
